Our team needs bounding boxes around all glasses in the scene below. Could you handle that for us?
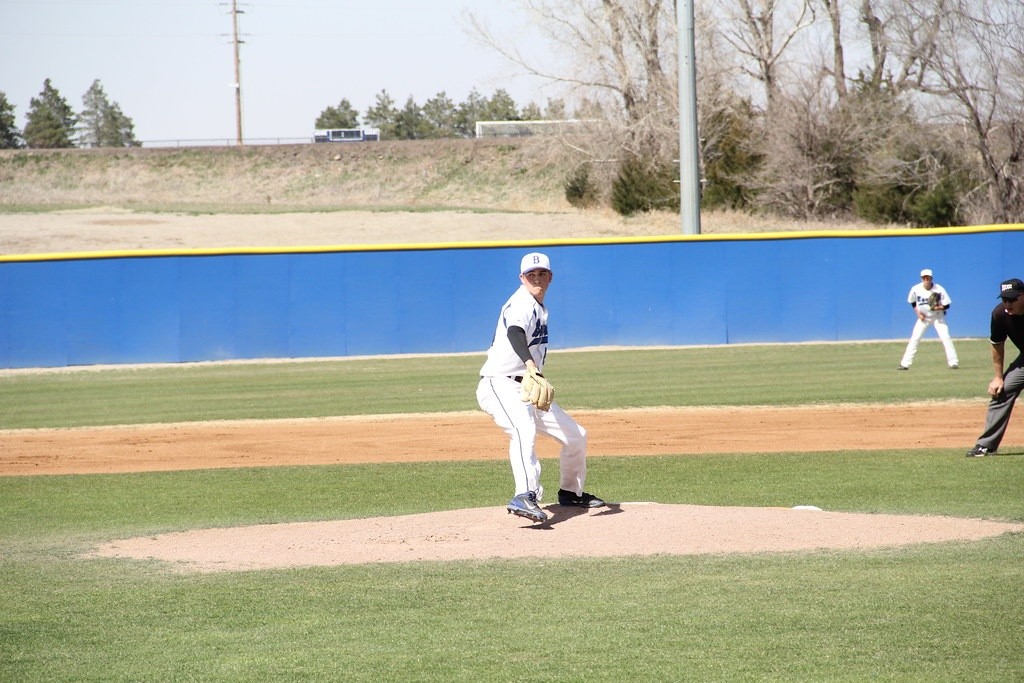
[1001,294,1024,304]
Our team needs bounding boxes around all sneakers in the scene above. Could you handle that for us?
[966,444,996,457]
[506,489,548,523]
[558,489,604,508]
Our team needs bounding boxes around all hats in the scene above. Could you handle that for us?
[521,252,551,274]
[920,269,933,278]
[996,279,1024,299]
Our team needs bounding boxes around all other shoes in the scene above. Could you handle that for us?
[950,365,958,369]
[897,364,908,370]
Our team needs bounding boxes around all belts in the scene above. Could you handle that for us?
[508,375,524,384]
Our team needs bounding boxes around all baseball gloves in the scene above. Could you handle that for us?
[520,366,556,412]
[928,292,941,311]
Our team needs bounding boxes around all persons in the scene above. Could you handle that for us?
[476,252,604,522]
[897,269,959,369]
[967,278,1024,456]
